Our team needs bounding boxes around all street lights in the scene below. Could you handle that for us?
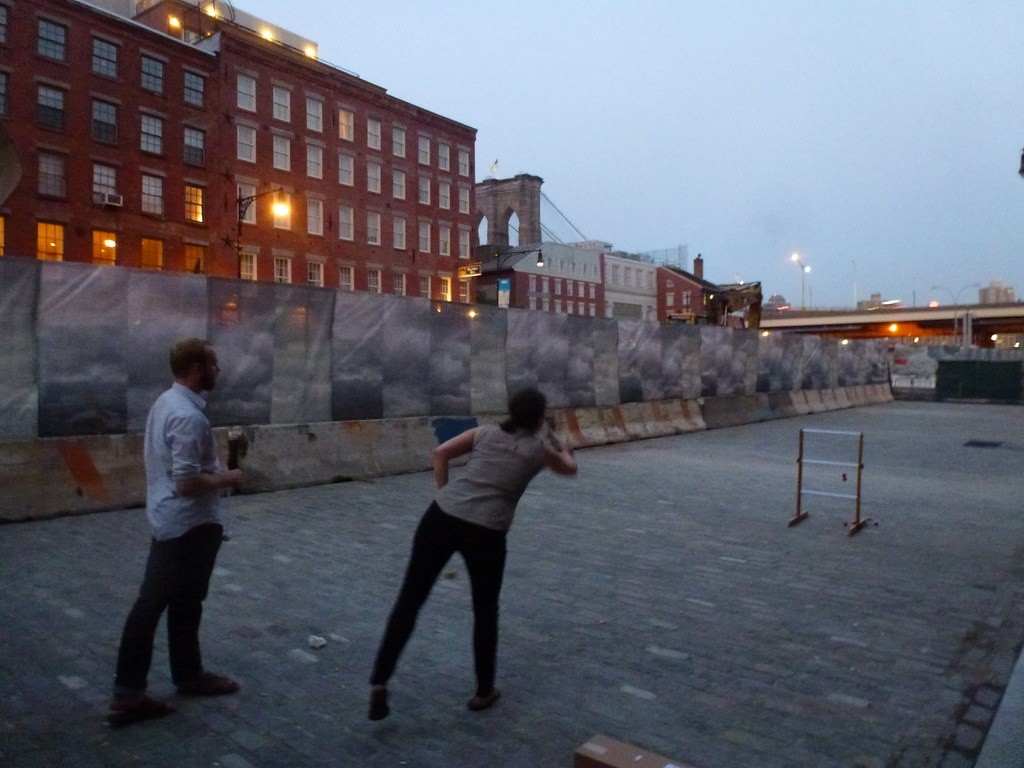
[790,251,810,313]
[234,185,292,280]
[494,246,544,308]
[930,282,981,337]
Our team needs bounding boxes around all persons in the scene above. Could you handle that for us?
[107,337,243,724]
[369,387,578,723]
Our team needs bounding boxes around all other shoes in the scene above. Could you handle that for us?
[367,686,391,720]
[107,690,181,723]
[467,685,502,711]
[172,670,244,698]
